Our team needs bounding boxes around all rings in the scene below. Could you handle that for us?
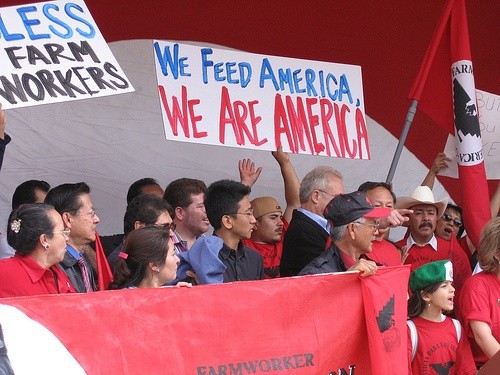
[441,161,443,164]
[441,165,442,168]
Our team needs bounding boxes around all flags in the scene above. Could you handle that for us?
[405,0,493,256]
[94,230,113,292]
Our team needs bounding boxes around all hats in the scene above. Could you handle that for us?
[250,197,282,219]
[409,260,453,292]
[323,191,393,227]
[394,186,448,220]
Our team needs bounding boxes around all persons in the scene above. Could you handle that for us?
[395,186,473,319]
[12,180,51,210]
[279,165,346,278]
[204,180,265,283]
[0,104,12,174]
[126,177,165,203]
[326,181,414,266]
[407,260,479,375]
[0,202,77,297]
[42,182,100,293]
[163,177,210,253]
[421,152,500,261]
[238,146,301,279]
[456,215,500,369]
[107,194,176,286]
[106,226,200,289]
[298,191,391,275]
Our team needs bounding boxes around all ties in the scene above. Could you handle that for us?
[78,257,93,292]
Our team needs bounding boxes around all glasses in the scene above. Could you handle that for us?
[441,213,462,227]
[153,222,176,232]
[236,208,253,215]
[52,228,70,236]
[78,211,95,217]
